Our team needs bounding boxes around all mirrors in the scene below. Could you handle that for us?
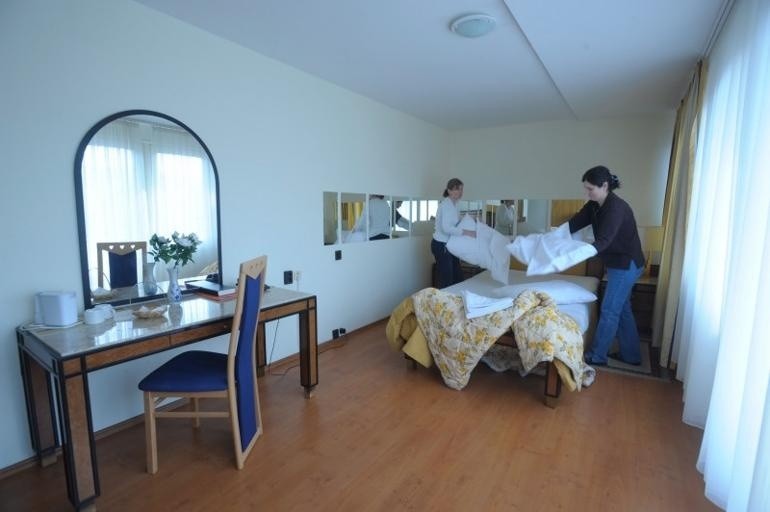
[460,196,550,237]
[321,190,439,247]
[73,108,225,314]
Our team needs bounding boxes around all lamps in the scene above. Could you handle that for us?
[636,227,664,280]
[449,12,499,41]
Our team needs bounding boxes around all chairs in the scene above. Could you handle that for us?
[136,255,272,474]
[93,240,148,293]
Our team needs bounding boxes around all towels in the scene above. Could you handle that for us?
[459,290,516,320]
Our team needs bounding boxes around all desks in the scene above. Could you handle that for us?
[12,278,322,511]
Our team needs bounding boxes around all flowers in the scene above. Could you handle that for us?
[167,231,204,270]
[145,234,172,264]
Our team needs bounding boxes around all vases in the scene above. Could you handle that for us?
[144,263,157,293]
[168,269,183,306]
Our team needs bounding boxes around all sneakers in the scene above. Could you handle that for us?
[608,352,641,366]
[584,358,608,366]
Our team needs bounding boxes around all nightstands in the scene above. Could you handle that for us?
[600,273,659,342]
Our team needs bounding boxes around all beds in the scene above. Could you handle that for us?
[397,241,605,411]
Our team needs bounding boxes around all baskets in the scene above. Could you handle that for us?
[129,281,167,318]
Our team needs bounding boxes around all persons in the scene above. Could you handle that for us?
[431,178,476,288]
[494,200,526,236]
[351,194,390,240]
[388,201,413,232]
[568,165,645,366]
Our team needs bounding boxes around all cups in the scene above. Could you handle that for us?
[84,303,117,325]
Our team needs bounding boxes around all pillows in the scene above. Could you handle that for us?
[445,215,512,286]
[492,279,600,306]
[509,223,599,277]
